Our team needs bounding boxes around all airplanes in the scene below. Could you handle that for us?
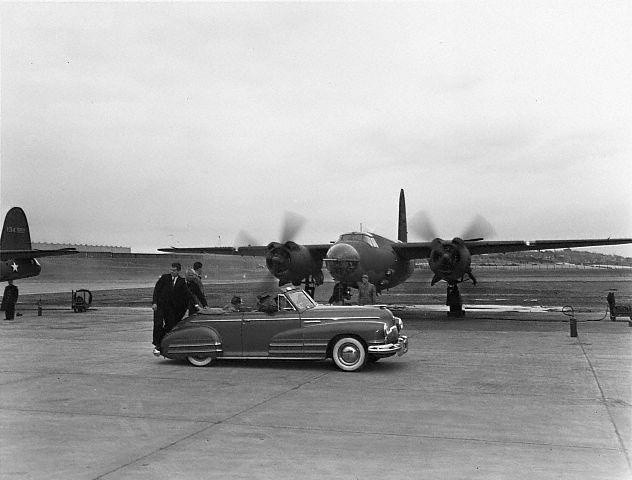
[156,187,632,306]
[0,206,80,283]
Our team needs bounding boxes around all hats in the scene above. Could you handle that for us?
[362,275,368,277]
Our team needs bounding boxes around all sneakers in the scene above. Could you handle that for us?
[153,347,160,356]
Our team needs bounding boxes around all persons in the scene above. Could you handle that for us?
[151,262,205,356]
[356,274,378,306]
[329,277,349,305]
[184,262,209,318]
[256,292,278,313]
[273,294,287,310]
[0,280,19,321]
[226,296,244,312]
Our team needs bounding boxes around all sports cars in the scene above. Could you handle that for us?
[155,283,409,373]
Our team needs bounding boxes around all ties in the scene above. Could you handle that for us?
[172,277,175,286]
